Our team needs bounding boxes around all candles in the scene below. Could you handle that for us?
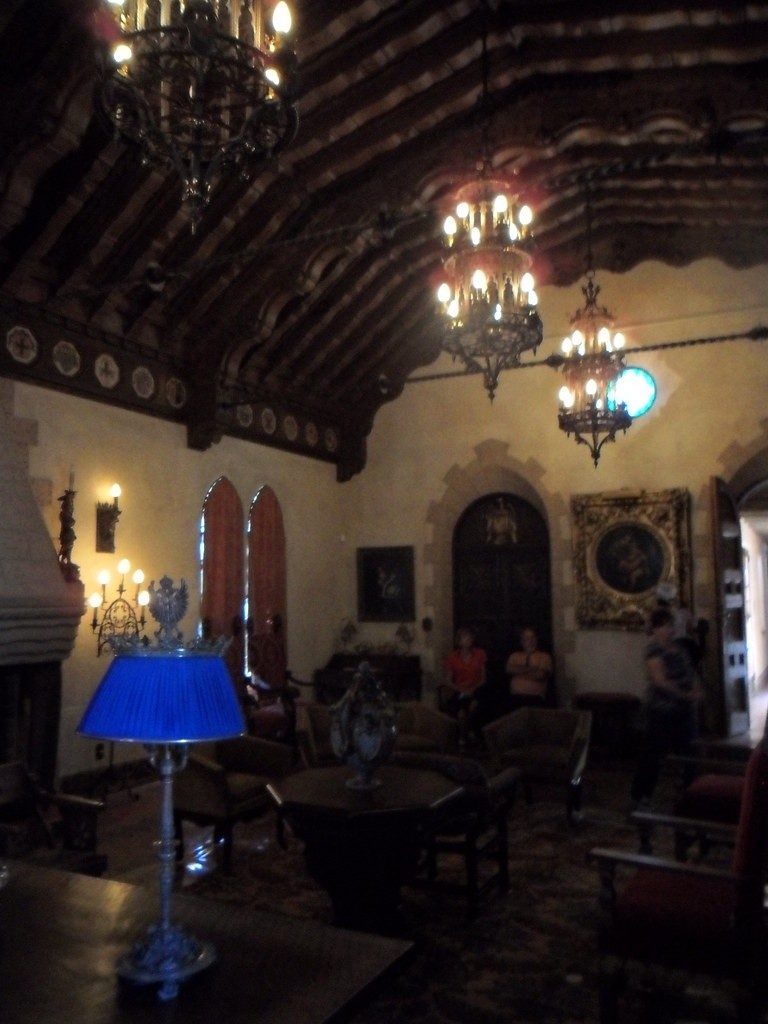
[69,463,75,490]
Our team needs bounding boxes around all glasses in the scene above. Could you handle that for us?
[526,655,530,667]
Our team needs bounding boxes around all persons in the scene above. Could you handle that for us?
[673,618,709,675]
[445,627,488,718]
[626,609,699,823]
[506,628,551,712]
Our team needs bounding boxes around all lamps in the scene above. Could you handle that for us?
[86,0,304,236]
[556,175,633,468]
[73,558,249,984]
[396,614,418,654]
[97,483,122,553]
[330,659,404,790]
[335,615,359,655]
[434,157,543,404]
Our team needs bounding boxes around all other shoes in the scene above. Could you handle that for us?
[631,796,658,812]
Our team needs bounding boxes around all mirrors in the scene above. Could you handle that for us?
[246,483,288,672]
[197,475,243,680]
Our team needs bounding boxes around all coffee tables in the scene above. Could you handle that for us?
[265,752,522,934]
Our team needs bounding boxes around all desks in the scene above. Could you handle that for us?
[0,856,412,1024]
[262,760,464,885]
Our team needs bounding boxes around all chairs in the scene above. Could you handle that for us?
[482,707,592,822]
[667,740,745,863]
[144,735,291,873]
[0,762,107,876]
[307,703,459,782]
[584,737,768,1024]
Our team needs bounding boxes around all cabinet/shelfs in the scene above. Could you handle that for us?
[313,653,423,703]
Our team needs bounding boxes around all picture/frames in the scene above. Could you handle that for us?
[357,544,416,623]
[570,488,694,635]
